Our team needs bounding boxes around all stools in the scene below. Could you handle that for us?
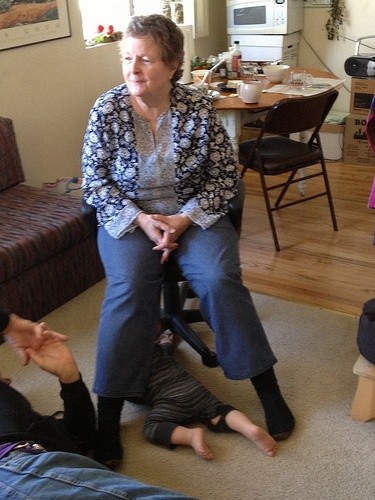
[351,355,375,422]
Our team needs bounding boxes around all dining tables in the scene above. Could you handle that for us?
[211,67,344,197]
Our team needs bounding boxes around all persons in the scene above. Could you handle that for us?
[0,307,200,500]
[81,13,296,469]
[143,334,278,460]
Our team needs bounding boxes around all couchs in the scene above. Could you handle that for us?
[0,117,106,347]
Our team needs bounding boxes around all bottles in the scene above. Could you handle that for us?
[218,40,243,77]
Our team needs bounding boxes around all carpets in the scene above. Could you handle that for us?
[0,278,375,500]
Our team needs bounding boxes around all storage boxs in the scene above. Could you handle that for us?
[309,123,345,160]
[240,118,274,141]
[231,33,300,68]
[349,76,375,115]
[342,114,375,167]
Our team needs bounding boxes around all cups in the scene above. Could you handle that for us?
[191,69,212,85]
[291,70,313,89]
[236,80,262,104]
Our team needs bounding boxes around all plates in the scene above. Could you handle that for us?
[217,82,236,91]
[208,90,220,101]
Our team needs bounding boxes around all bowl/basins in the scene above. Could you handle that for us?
[263,65,290,82]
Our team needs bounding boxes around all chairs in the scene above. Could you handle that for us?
[156,179,246,368]
[239,89,339,251]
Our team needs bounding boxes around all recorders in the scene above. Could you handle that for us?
[345,53,375,77]
